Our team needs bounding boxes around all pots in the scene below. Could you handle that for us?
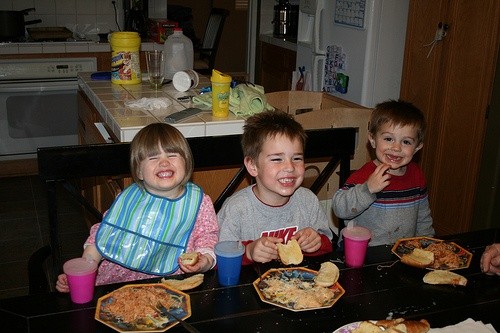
[0,11,41,41]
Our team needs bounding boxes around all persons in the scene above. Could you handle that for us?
[332,97,436,246]
[215,107,334,265]
[480,243,500,276]
[56,122,219,293]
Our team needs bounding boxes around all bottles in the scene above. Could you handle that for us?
[111,31,142,84]
[162,28,193,82]
[210,70,232,120]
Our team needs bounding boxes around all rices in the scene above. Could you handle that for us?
[263,277,335,309]
[107,290,181,322]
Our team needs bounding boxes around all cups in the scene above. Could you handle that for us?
[145,50,166,86]
[213,241,245,287]
[63,258,98,305]
[173,71,198,91]
[343,227,370,267]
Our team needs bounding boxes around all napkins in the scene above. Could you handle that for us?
[428,318,497,333]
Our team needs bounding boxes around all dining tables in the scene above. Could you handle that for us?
[0,228,500,333]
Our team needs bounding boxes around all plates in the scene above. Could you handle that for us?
[27,27,73,40]
[391,236,472,270]
[93,283,191,333]
[254,267,344,311]
[334,320,384,333]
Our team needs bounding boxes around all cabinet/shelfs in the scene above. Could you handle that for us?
[74,85,133,231]
[399,0,500,234]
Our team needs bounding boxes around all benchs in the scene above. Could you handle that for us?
[37,126,358,285]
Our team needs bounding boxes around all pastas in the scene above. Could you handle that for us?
[408,240,464,267]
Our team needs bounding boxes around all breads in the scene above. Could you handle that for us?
[423,269,467,285]
[180,252,198,265]
[400,248,435,268]
[162,274,204,290]
[314,262,340,286]
[352,317,430,333]
[276,239,304,265]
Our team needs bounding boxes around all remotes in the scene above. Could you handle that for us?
[164,107,203,124]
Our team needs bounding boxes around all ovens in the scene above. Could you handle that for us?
[0,56,98,160]
